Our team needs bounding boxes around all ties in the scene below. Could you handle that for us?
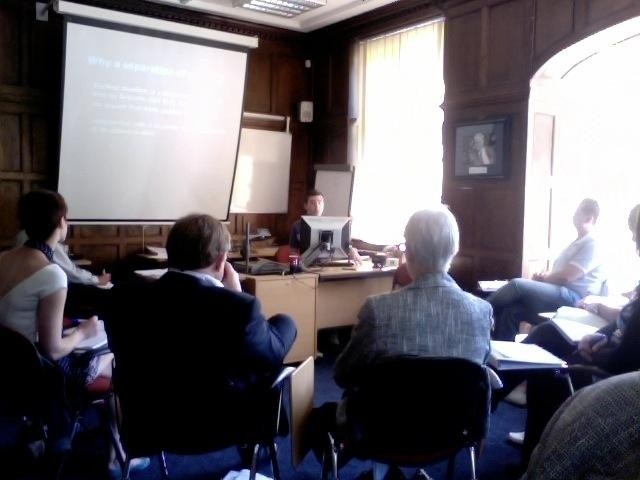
[480,149,483,162]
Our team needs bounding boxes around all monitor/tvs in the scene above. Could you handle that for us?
[298,214,349,263]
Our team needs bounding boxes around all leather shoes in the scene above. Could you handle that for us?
[244,442,276,467]
[510,431,524,442]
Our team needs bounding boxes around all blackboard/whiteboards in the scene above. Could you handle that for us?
[230,128,293,213]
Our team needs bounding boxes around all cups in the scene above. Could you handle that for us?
[289,255,299,271]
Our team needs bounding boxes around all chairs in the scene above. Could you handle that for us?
[320,355,492,480]
[553,364,616,397]
[0,325,118,480]
[103,355,295,480]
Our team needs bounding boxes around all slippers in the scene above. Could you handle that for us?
[111,457,151,479]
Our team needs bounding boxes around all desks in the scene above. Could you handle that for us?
[137,246,279,270]
[236,256,400,364]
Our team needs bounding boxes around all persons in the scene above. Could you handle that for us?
[451,123,506,183]
[481,198,609,343]
[288,191,364,267]
[489,203,640,448]
[16,186,112,287]
[333,207,493,419]
[104,213,300,456]
[1,188,103,362]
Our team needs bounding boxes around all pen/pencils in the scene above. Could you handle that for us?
[102,268,105,275]
[72,319,88,322]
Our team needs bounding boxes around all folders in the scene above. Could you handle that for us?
[489,339,569,370]
